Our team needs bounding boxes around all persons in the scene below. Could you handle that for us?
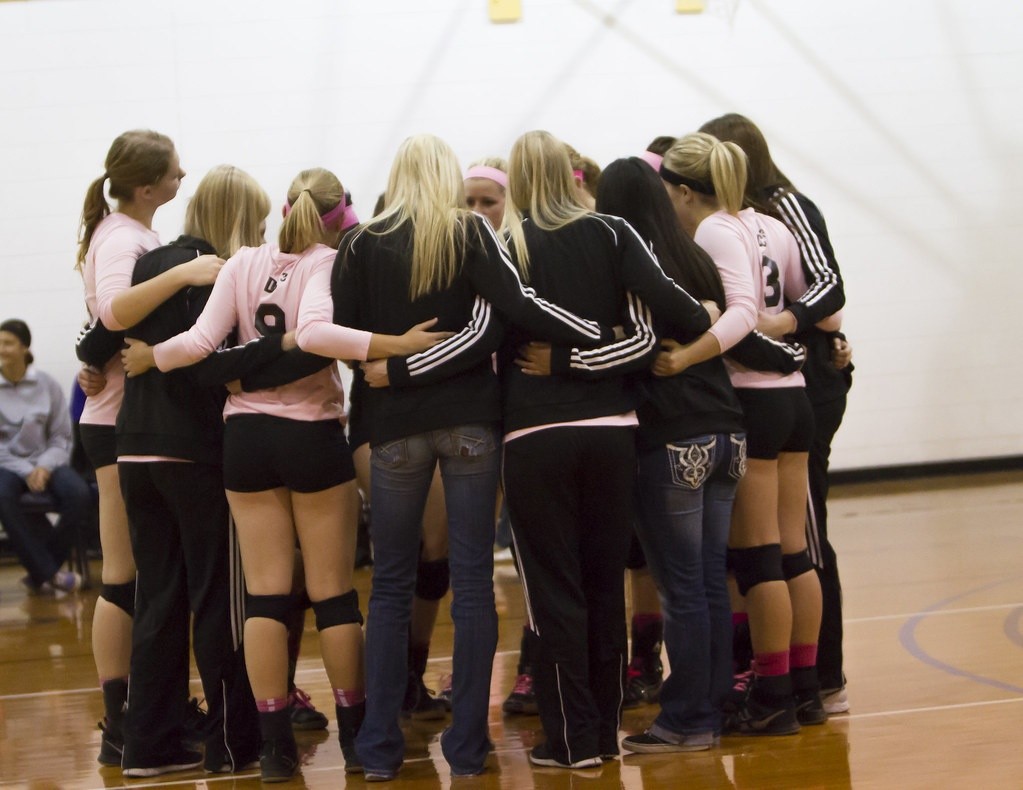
[122,166,457,782]
[0,319,89,593]
[512,156,808,753]
[73,129,228,769]
[650,133,843,737]
[77,128,852,730]
[74,162,297,778]
[359,131,719,769]
[699,114,855,715]
[225,130,629,781]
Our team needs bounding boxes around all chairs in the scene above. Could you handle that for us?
[0,494,89,589]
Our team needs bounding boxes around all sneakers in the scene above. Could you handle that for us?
[795,694,828,725]
[623,729,711,752]
[623,665,664,709]
[503,672,543,715]
[721,707,801,735]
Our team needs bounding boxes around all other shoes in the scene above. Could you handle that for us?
[821,687,851,713]
[184,698,208,739]
[289,689,329,729]
[256,742,299,782]
[340,735,364,773]
[96,723,123,765]
[402,684,446,720]
[63,570,82,593]
[120,742,203,776]
[530,742,604,768]
[438,680,454,708]
[733,669,754,694]
[202,737,259,773]
[364,764,404,782]
[22,577,43,598]
